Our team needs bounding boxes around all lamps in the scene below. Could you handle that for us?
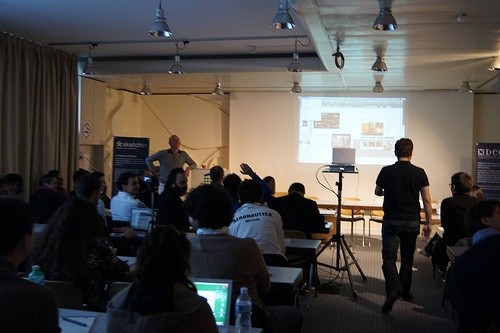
[147,0,172,38]
[212,82,226,97]
[83,43,96,75]
[287,37,303,72]
[373,82,384,93]
[271,0,296,30]
[291,82,302,93]
[458,81,472,96]
[168,42,185,75]
[372,0,398,31]
[372,56,389,71]
[140,80,153,96]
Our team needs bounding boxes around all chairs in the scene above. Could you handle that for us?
[273,191,442,248]
[283,230,320,297]
[23,278,84,309]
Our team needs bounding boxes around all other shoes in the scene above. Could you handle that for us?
[382,292,398,314]
[399,292,412,301]
[433,271,445,280]
[419,249,431,257]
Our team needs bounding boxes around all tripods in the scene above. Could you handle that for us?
[316,171,366,300]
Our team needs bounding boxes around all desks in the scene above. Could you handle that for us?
[57,308,264,333]
[31,223,322,294]
[117,256,304,307]
[317,200,436,214]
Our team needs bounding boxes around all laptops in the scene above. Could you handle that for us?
[329,148,356,167]
[184,276,232,332]
[130,207,154,237]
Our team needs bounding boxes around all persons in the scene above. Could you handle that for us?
[433,171,500,333]
[0,199,63,333]
[108,172,149,226]
[188,183,271,302]
[146,135,197,196]
[0,162,323,333]
[374,138,433,313]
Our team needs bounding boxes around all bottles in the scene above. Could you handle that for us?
[28,265,45,287]
[436,200,441,214]
[235,287,253,333]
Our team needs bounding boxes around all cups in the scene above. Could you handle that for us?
[201,164,206,168]
